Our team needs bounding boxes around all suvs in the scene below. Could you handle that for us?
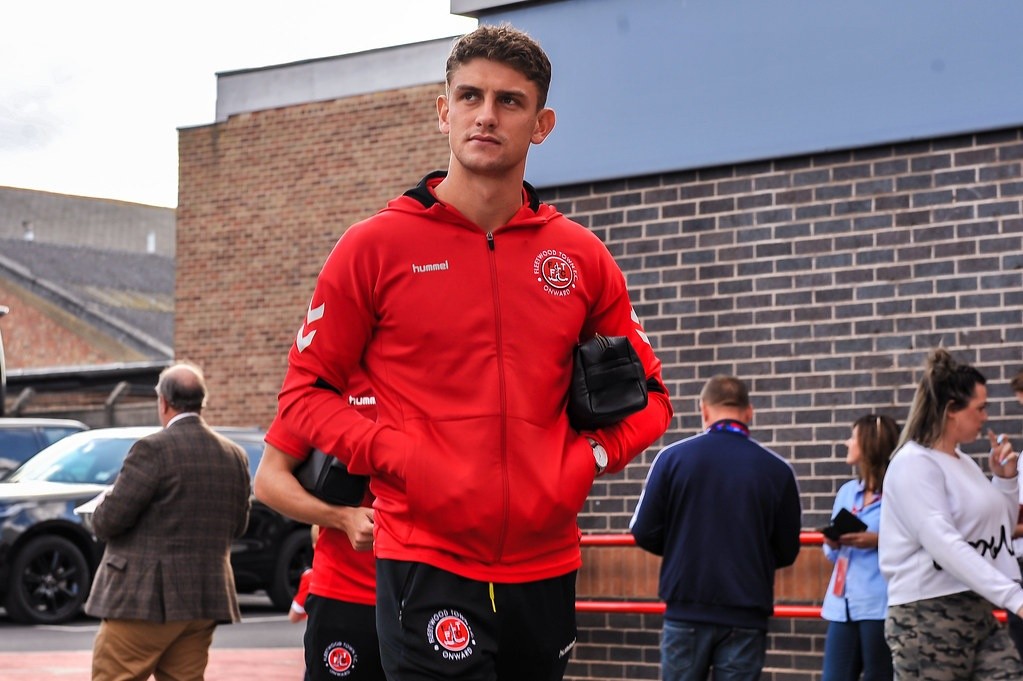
[0,425,315,626]
[1,414,98,483]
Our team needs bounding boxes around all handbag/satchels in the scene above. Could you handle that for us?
[291,444,370,508]
[566,332,649,432]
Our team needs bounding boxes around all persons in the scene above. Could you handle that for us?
[628,377,801,681]
[820,347,1023,681]
[78,364,252,680]
[253,26,674,680]
